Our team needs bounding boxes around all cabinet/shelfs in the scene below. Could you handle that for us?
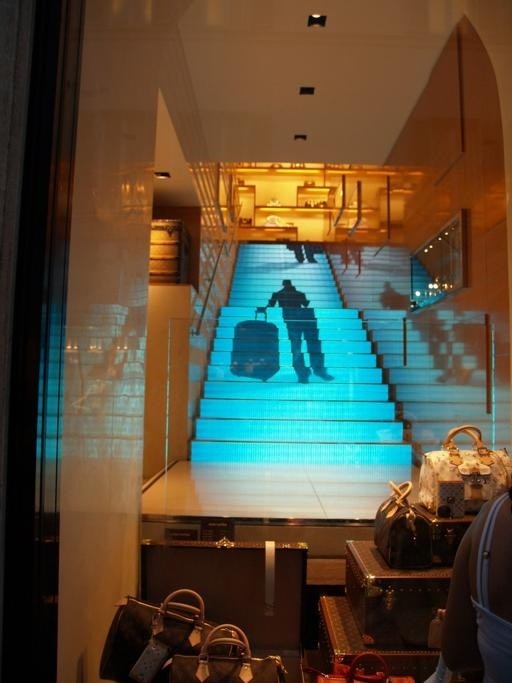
[233,162,336,240]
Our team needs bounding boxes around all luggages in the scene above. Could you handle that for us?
[409,501,481,566]
[317,595,445,683]
[229,308,280,381]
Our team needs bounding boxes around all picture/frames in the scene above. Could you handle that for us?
[407,206,469,309]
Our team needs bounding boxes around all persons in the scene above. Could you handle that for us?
[441,471,511,683]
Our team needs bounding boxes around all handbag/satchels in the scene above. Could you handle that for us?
[417,423,512,513]
[437,480,466,518]
[317,652,418,683]
[98,586,289,683]
[373,479,429,569]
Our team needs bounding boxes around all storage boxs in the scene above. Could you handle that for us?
[410,499,475,561]
[134,531,312,681]
[313,589,459,682]
[149,215,193,285]
